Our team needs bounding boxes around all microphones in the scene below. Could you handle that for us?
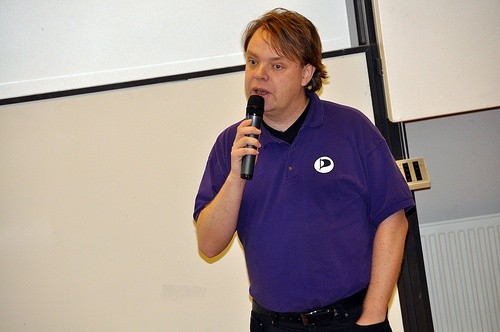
[240,95,264,180]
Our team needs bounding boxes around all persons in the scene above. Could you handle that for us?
[193,5,415,332]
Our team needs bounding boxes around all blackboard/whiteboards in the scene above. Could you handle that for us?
[374,0,500,122]
[0,54,404,332]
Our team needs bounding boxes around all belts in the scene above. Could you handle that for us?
[252,299,360,325]
